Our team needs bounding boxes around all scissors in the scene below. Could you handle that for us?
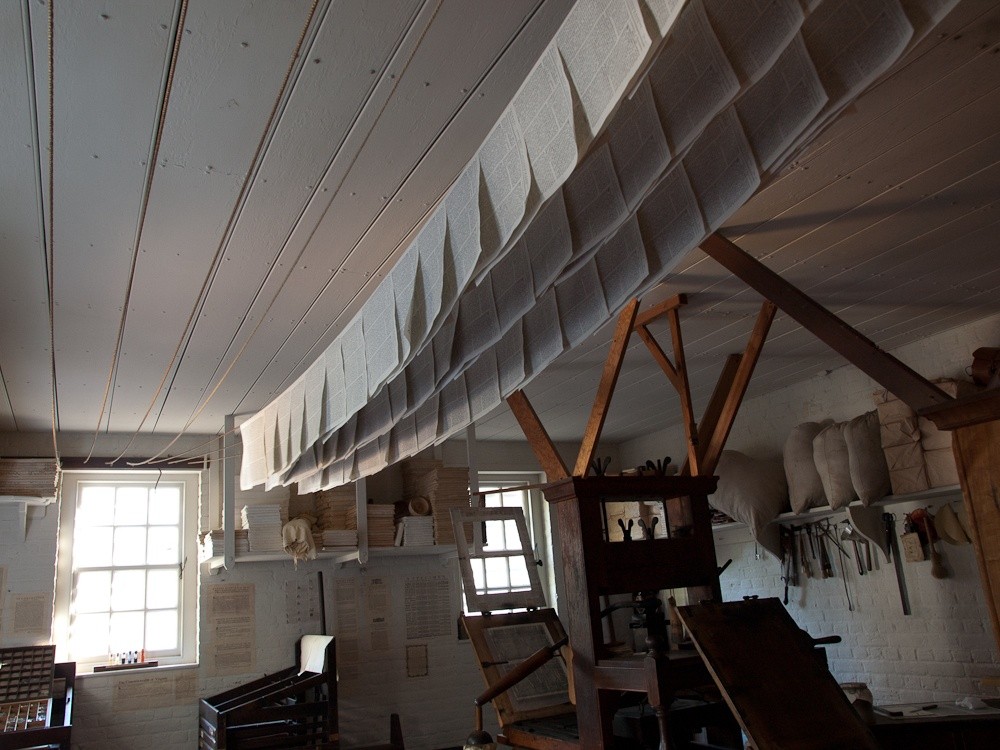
[880,513,893,556]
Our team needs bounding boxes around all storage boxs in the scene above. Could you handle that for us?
[872,390,959,495]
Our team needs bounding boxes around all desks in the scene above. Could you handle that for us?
[874,697,1000,750]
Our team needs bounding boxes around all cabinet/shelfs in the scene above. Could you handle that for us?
[541,474,744,750]
[920,391,999,649]
